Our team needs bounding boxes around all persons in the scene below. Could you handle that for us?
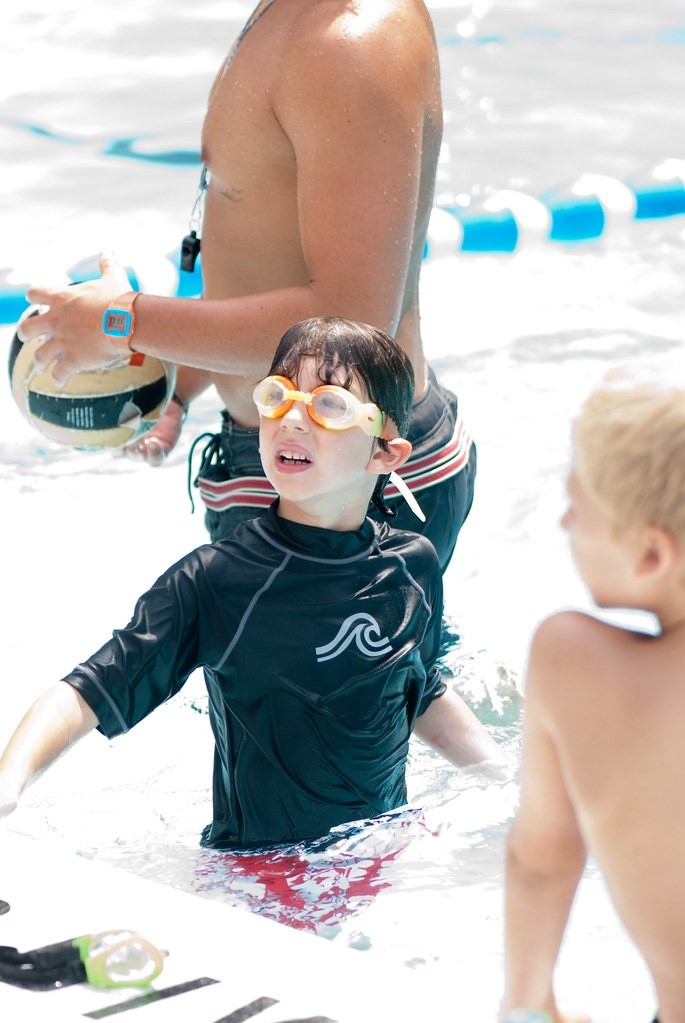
[499,362,684,1023]
[15,0,499,769]
[0,313,509,851]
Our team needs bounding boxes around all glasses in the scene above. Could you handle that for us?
[252,375,401,442]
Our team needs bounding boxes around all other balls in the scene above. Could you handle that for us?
[8,275,177,449]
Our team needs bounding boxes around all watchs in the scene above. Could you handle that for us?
[100,290,141,356]
